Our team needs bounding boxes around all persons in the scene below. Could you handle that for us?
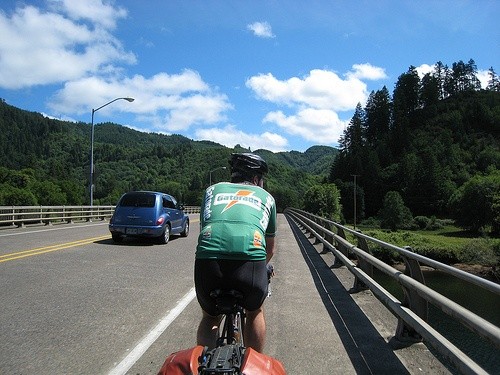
[193,152,277,352]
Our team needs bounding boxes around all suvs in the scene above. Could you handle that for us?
[108,190,190,244]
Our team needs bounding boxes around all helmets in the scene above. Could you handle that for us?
[227,150,268,176]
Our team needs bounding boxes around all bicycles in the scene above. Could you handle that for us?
[157,263,287,375]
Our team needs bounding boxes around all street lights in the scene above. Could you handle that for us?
[90,97,135,206]
[210,167,226,186]
